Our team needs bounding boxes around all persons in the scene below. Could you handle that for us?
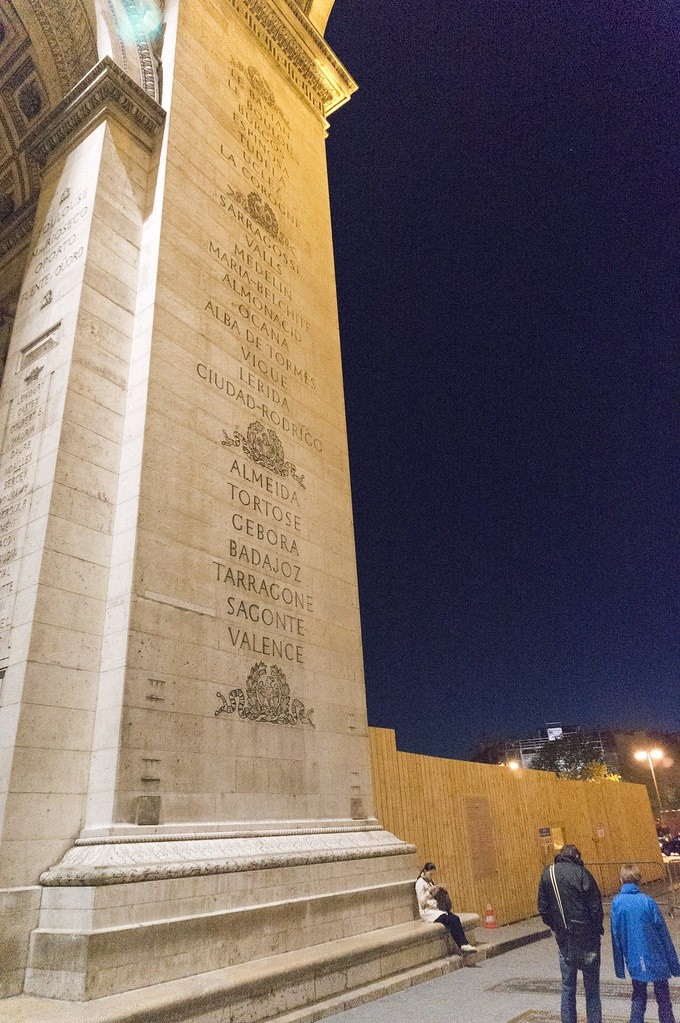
[536,843,607,1023]
[413,861,479,958]
[609,861,680,1023]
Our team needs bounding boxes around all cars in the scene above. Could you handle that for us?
[663,834,680,855]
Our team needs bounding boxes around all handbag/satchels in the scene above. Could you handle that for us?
[564,944,597,973]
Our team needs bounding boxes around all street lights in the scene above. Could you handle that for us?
[634,749,665,826]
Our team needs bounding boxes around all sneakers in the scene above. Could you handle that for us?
[460,944,479,953]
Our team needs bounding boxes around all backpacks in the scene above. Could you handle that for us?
[433,888,452,911]
